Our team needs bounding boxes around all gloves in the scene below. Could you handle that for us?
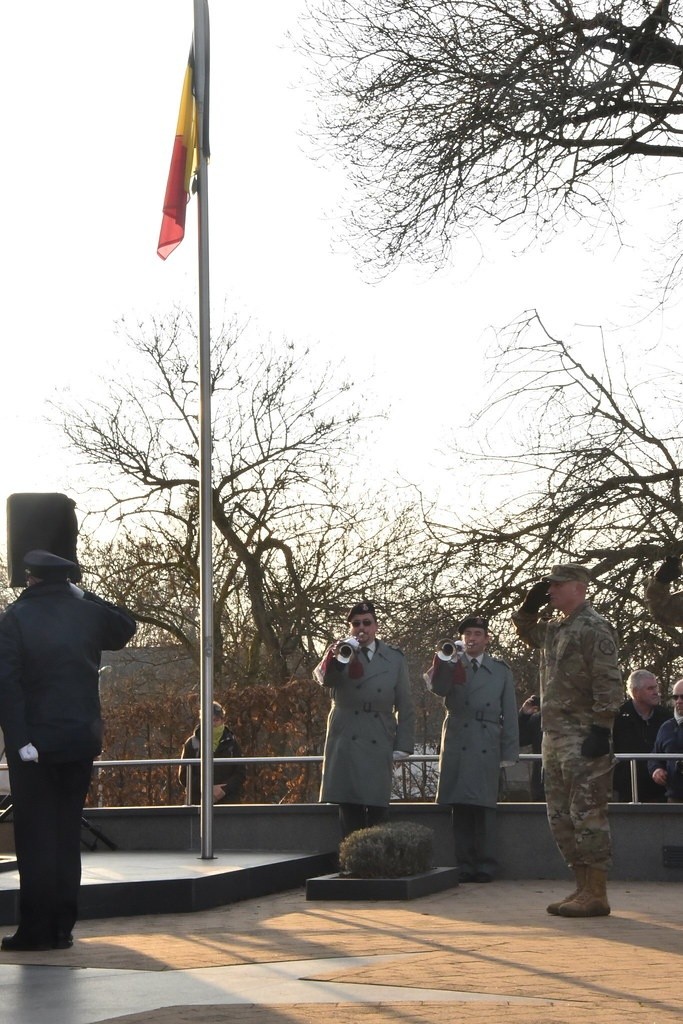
[581,725,610,758]
[393,750,411,761]
[498,760,516,768]
[68,580,84,601]
[454,640,465,650]
[343,638,359,651]
[18,742,39,764]
[522,582,552,613]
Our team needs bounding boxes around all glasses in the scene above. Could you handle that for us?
[671,694,683,700]
[350,620,372,628]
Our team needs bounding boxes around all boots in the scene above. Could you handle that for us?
[546,865,585,915]
[558,867,610,918]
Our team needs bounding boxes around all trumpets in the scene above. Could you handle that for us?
[436,637,473,661]
[334,631,364,665]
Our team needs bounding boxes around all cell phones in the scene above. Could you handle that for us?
[531,696,541,706]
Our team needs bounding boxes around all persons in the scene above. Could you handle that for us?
[179,701,247,805]
[646,679,683,804]
[0,549,135,950]
[612,669,674,803]
[511,563,624,916]
[426,617,519,884]
[313,601,417,842]
[518,694,545,801]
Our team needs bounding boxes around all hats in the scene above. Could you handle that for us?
[200,701,225,717]
[542,563,591,582]
[457,617,488,633]
[22,548,75,579]
[347,602,375,622]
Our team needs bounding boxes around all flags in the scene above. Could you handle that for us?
[156,32,211,261]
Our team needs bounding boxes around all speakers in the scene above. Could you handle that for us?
[6,493,82,588]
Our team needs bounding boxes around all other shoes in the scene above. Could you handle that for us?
[1,935,56,950]
[457,871,473,883]
[53,934,74,949]
[474,872,497,882]
